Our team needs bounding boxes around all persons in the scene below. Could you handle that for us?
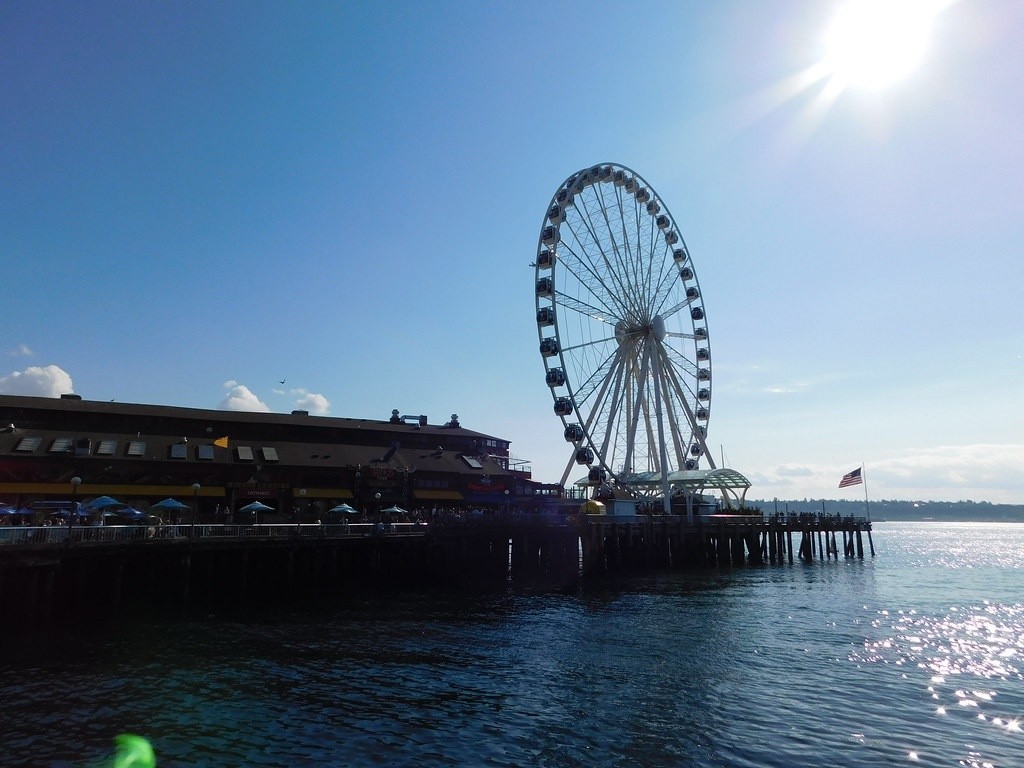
[412,503,542,528]
[0,515,352,546]
[716,502,856,527]
[374,513,410,532]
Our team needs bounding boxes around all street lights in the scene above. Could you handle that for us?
[190,484,201,537]
[67,477,81,540]
[296,489,306,536]
[373,493,381,538]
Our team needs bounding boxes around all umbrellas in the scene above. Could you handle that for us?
[83,495,126,526]
[118,507,144,521]
[240,500,275,524]
[329,502,360,514]
[17,505,34,520]
[147,499,190,537]
[0,504,17,515]
[380,504,409,514]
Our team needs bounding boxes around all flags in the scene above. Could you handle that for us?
[838,467,862,489]
